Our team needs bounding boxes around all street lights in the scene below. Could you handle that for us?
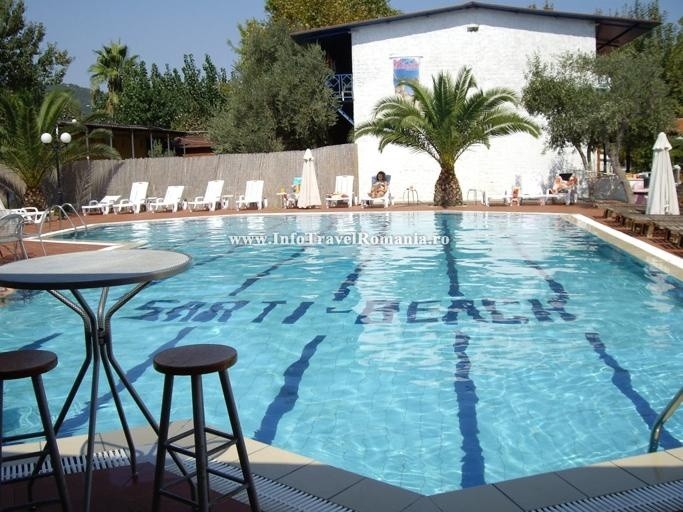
[41,127,72,224]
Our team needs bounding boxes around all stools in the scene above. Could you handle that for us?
[0,349,73,512]
[151,344,260,512]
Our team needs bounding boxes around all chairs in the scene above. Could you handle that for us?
[596,203,683,244]
[14,208,49,262]
[0,214,24,261]
[0,199,54,223]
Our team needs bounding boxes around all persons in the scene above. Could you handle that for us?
[368,171,388,199]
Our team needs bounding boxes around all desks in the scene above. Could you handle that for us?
[0,250,191,512]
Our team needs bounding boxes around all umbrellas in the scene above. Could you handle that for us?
[644,131,681,216]
[297,148,322,209]
